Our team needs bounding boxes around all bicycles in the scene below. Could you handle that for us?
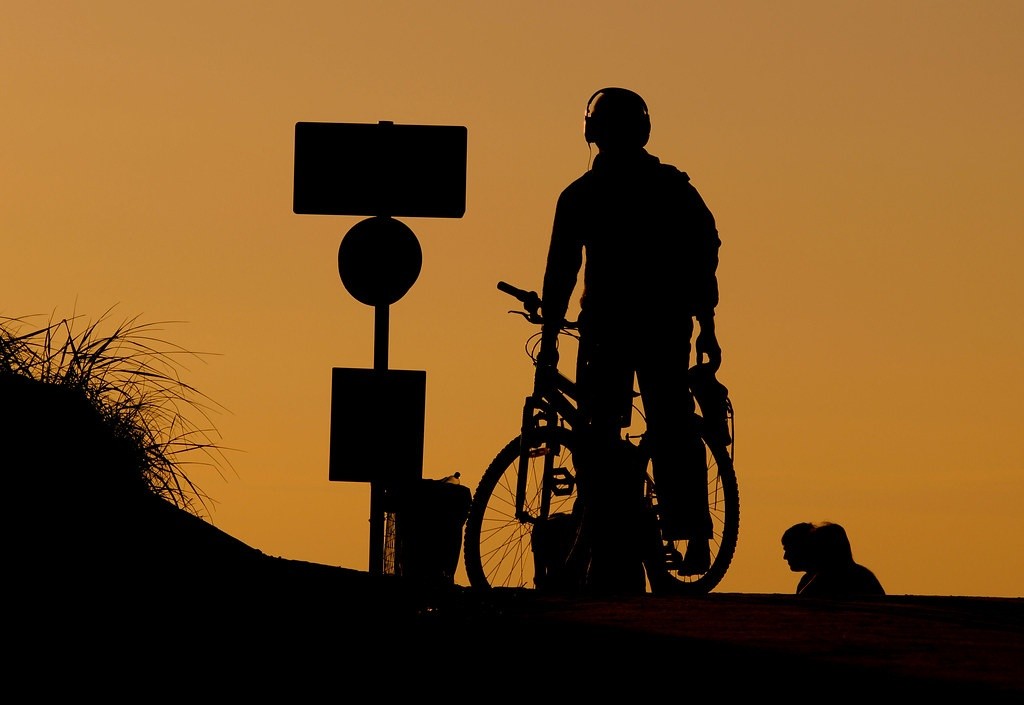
[464,280,741,597]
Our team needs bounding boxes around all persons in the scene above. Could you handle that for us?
[781,522,886,595]
[535,87,722,576]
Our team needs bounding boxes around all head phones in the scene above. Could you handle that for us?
[584,87,651,148]
